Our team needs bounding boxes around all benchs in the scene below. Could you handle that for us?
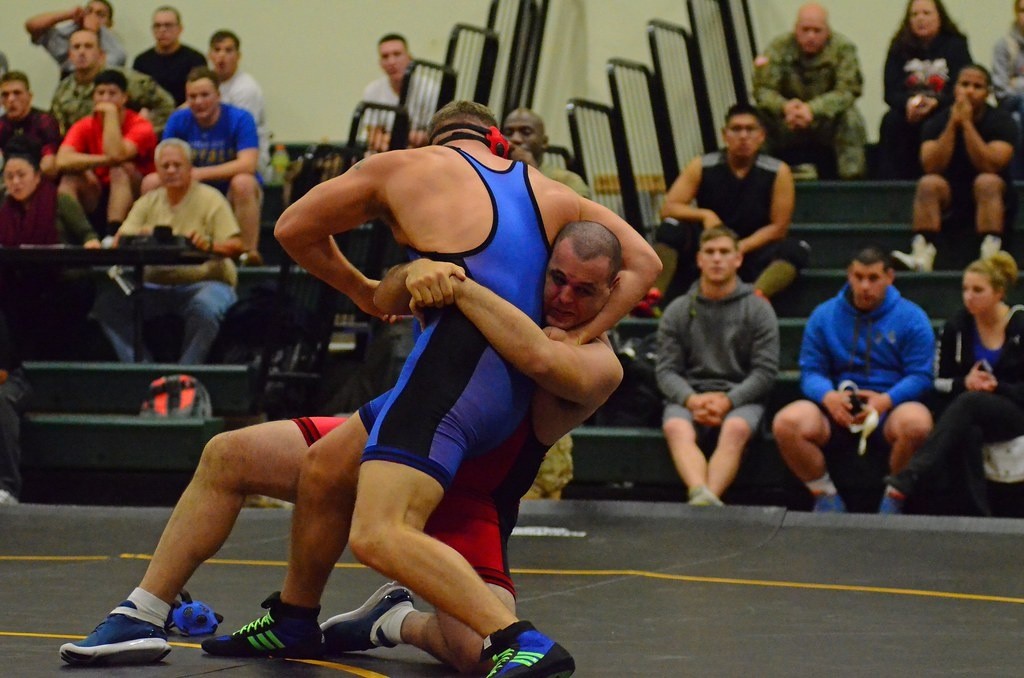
[569,143,1023,515]
[0,142,371,505]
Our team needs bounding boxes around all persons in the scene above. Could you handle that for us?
[879,0,973,179]
[141,67,263,267]
[894,63,1016,271]
[991,0,1024,151]
[25,0,208,135]
[0,154,100,363]
[0,255,34,505]
[94,137,245,397]
[209,31,270,173]
[55,70,157,247]
[881,250,1024,516]
[59,218,624,678]
[752,3,868,180]
[772,245,935,514]
[633,105,795,317]
[363,33,439,153]
[505,108,595,201]
[0,70,60,186]
[654,225,781,507]
[202,100,664,678]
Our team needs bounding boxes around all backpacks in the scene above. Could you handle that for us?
[138,374,213,419]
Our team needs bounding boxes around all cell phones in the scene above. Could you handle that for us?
[978,359,992,374]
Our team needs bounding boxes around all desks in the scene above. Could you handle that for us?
[1,242,192,364]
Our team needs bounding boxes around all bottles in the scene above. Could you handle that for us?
[272,146,289,184]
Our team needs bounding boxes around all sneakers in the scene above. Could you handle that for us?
[319,580,415,653]
[201,591,327,659]
[791,164,819,182]
[479,620,575,678]
[981,235,1002,259]
[812,492,847,513]
[880,495,901,515]
[889,234,937,272]
[59,613,172,666]
